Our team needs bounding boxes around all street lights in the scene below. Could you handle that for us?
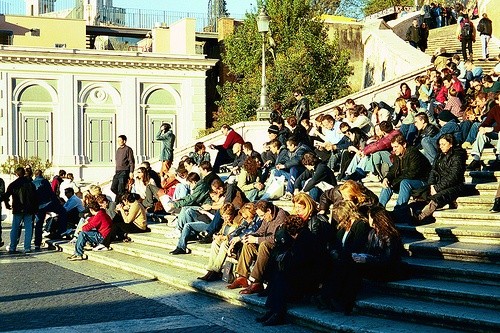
[256,8,272,122]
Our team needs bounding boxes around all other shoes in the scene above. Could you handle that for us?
[9,250,17,253]
[465,160,479,171]
[486,54,489,58]
[257,312,273,322]
[35,247,42,252]
[263,313,286,325]
[94,244,109,251]
[70,238,78,243]
[197,271,220,281]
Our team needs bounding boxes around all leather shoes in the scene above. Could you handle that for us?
[169,248,185,255]
[227,278,249,289]
[240,283,263,295]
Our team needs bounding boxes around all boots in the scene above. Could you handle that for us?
[489,198,500,213]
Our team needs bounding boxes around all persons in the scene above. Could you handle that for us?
[269,99,469,225]
[489,183,500,213]
[94,13,100,25]
[197,137,405,325]
[406,3,479,53]
[208,123,245,172]
[458,15,476,62]
[267,90,309,125]
[0,168,147,260]
[135,141,246,255]
[397,47,500,171]
[477,13,493,61]
[156,123,175,176]
[111,135,135,205]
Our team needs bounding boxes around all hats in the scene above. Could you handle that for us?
[268,125,279,133]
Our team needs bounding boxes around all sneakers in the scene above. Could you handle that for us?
[68,255,83,261]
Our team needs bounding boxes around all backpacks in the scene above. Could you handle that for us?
[461,21,472,39]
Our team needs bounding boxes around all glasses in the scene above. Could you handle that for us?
[343,128,350,134]
